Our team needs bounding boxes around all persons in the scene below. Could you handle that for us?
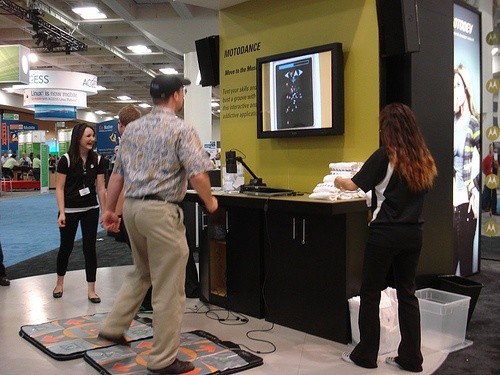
[53,124,109,304]
[33,154,40,180]
[481,148,494,212]
[29,153,34,162]
[0,241,11,286]
[48,154,57,166]
[98,75,218,375]
[115,106,156,313]
[19,152,32,179]
[2,154,19,179]
[1,153,8,162]
[454,69,482,277]
[334,104,437,373]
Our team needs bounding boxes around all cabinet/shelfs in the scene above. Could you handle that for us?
[181,186,374,346]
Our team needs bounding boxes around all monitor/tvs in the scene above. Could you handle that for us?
[256,42,345,138]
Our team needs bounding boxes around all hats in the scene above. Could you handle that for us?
[150,73,192,98]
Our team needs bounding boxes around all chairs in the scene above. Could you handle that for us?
[2,167,13,192]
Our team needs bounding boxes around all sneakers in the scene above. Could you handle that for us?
[341,351,377,368]
[386,357,402,368]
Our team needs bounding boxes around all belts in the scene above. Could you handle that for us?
[137,194,177,205]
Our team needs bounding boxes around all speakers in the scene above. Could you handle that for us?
[376,0,420,58]
[194,35,219,87]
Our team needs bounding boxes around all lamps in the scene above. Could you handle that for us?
[30,25,73,56]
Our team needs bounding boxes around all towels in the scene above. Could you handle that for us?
[309,162,366,200]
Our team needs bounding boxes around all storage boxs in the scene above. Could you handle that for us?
[414,288,473,353]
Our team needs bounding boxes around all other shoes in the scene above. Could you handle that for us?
[0,278,10,286]
[88,292,101,303]
[99,332,131,346]
[147,358,195,374]
[53,285,63,298]
